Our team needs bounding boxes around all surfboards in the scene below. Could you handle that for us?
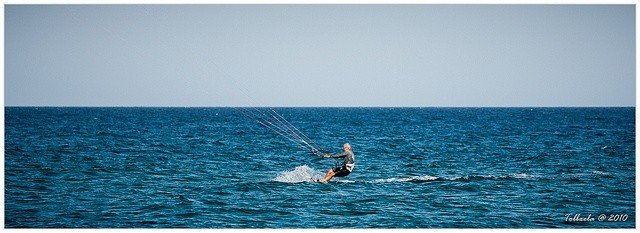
[309,178,329,184]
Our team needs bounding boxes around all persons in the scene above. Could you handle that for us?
[311,142,355,183]
